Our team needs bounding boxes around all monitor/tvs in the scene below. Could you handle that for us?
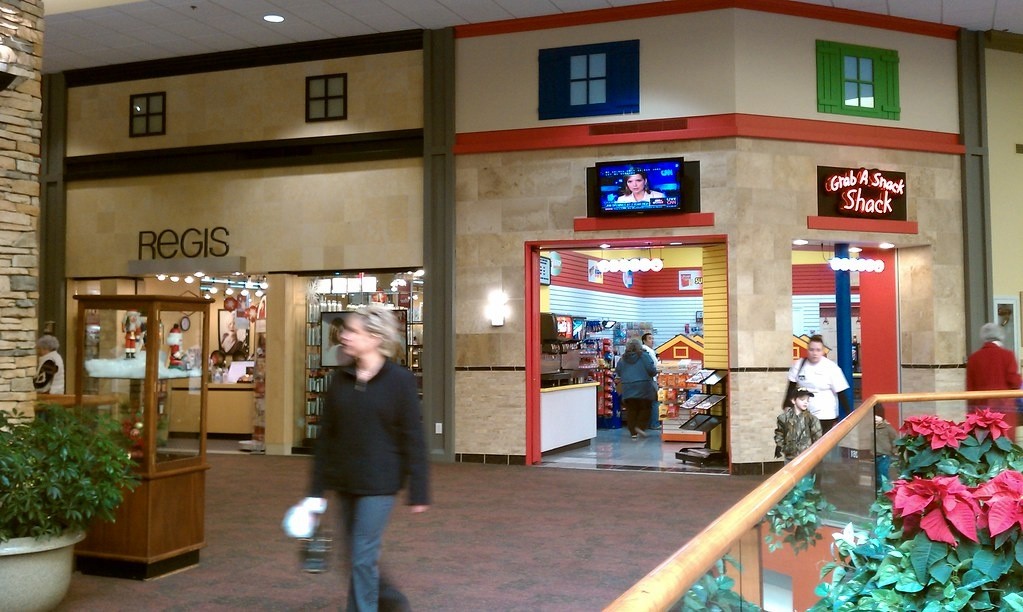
[593,157,686,216]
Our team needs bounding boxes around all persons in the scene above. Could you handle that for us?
[781,334,853,437]
[641,333,662,429]
[287,307,434,612]
[221,313,241,353]
[873,404,900,490]
[965,321,1022,446]
[616,339,659,438]
[121,308,142,359]
[615,167,665,201]
[33,335,66,394]
[773,386,823,478]
[210,349,226,368]
[325,316,354,366]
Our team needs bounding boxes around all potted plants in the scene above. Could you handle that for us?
[1,401,143,612]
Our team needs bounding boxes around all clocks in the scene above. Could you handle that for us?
[180,317,191,331]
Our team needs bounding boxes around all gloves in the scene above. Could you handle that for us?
[773,447,783,457]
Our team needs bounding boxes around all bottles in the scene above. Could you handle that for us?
[305,298,422,444]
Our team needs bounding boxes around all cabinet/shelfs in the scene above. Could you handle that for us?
[298,288,418,452]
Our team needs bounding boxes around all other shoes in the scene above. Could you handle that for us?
[634,426,648,439]
[299,559,329,574]
[630,433,637,437]
[303,540,332,553]
[651,425,663,430]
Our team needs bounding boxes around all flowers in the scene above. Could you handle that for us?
[803,410,1023,611]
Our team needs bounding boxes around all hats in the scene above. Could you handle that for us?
[873,403,884,419]
[790,388,815,398]
